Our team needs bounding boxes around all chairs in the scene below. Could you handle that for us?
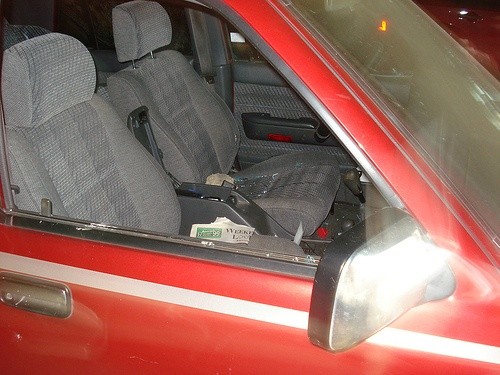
[0,1,340,256]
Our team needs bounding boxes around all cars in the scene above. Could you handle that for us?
[0,0,500,375]
